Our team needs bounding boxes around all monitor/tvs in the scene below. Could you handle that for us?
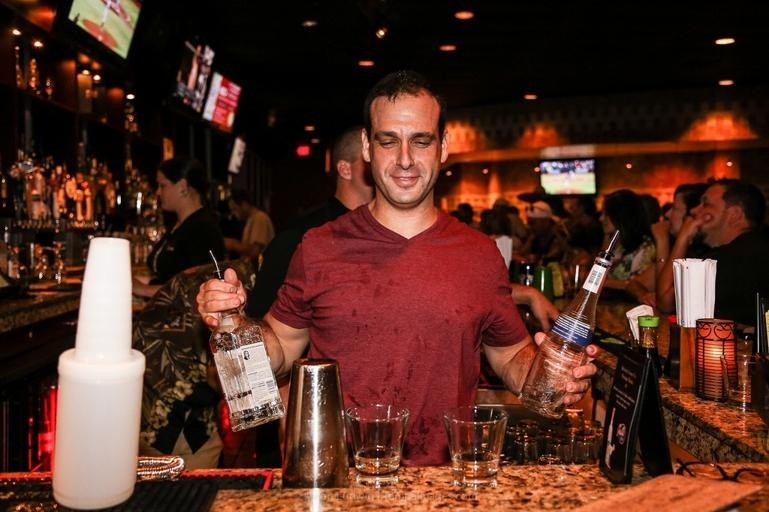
[538,158,598,198]
[63,1,146,67]
[172,38,218,115]
[201,71,246,137]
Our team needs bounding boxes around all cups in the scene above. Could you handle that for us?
[565,263,585,294]
[345,403,412,486]
[441,405,510,489]
[503,408,606,466]
[283,358,350,487]
[532,264,555,303]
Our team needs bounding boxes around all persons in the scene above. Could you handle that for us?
[450,177,767,359]
[193,69,603,471]
[132,154,226,299]
[222,192,276,266]
[244,128,562,467]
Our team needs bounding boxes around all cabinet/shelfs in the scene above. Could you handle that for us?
[1,4,134,333]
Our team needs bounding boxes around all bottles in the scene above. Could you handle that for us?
[200,250,287,434]
[637,315,660,352]
[3,155,157,282]
[51,236,147,511]
[522,226,624,417]
[521,265,531,320]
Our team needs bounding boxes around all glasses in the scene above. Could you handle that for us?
[524,205,549,215]
